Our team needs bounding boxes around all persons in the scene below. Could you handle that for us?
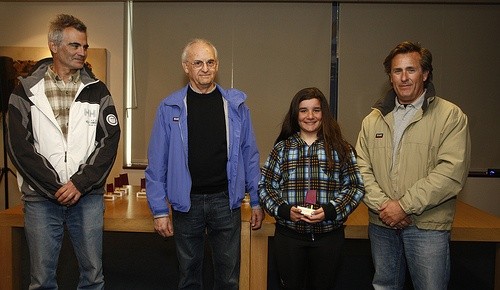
[144,38,264,290]
[257,87,366,290]
[354,41,472,290]
[5,14,121,290]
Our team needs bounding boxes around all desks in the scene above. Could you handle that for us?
[0,185,500,290]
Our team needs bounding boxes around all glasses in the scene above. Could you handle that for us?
[190,60,216,68]
